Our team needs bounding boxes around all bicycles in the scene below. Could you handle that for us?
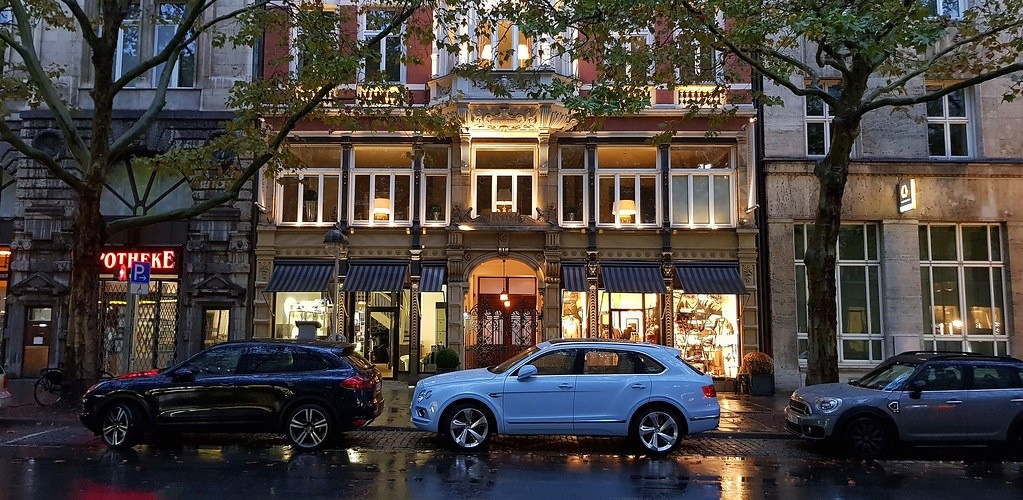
[34,361,115,407]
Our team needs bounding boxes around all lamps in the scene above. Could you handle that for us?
[497,189,513,212]
[611,200,636,223]
[482,45,493,65]
[518,44,529,68]
[374,197,390,220]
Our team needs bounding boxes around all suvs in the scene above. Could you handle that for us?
[78,338,385,453]
[783,350,1022,461]
[410,339,721,458]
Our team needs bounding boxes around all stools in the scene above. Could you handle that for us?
[735,373,753,396]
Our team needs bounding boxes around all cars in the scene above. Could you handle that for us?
[0,366,13,410]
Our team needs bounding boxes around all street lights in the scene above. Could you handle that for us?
[324,222,350,342]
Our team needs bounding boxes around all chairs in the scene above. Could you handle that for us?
[931,369,960,391]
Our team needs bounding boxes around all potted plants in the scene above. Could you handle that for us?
[436,348,461,375]
[741,351,775,395]
